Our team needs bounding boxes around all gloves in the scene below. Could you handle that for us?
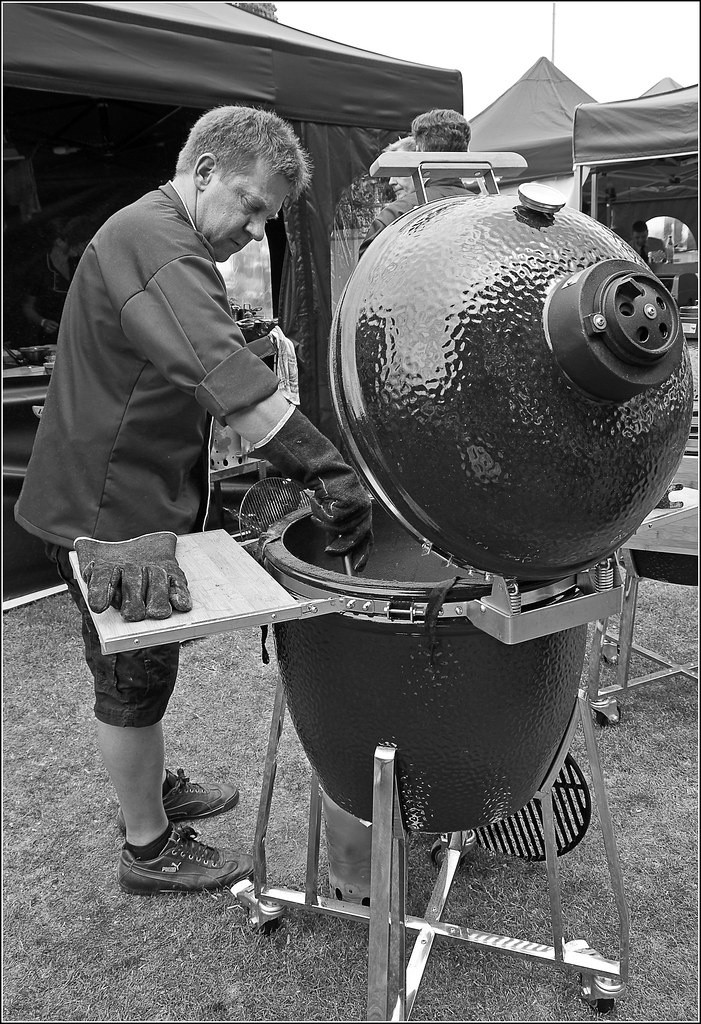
[252,409,377,574]
[74,529,190,622]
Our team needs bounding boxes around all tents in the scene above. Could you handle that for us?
[1,1,463,506]
[462,56,605,186]
[571,75,697,343]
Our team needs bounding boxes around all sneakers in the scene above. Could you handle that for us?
[118,823,256,892]
[115,778,240,826]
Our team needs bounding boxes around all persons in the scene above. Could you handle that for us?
[628,218,666,263]
[14,108,375,894]
[382,137,420,201]
[358,109,478,263]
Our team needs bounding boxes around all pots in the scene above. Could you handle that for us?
[258,150,696,839]
